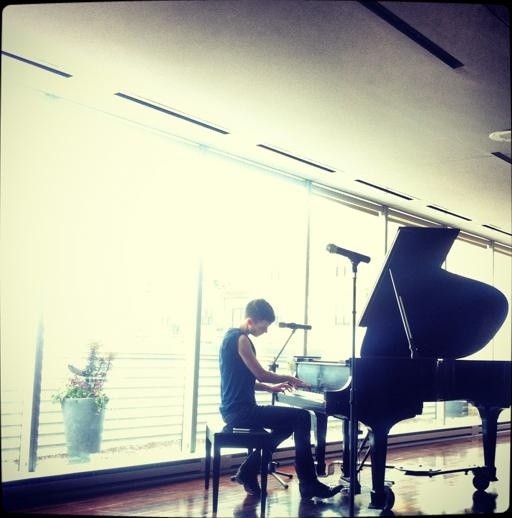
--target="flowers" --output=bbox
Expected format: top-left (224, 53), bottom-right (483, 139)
top-left (51, 340), bottom-right (114, 410)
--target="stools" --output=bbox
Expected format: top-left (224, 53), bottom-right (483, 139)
top-left (205, 425), bottom-right (271, 493)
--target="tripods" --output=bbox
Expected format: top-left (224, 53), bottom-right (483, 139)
top-left (231, 365), bottom-right (294, 489)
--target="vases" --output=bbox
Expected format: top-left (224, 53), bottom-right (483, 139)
top-left (61, 397), bottom-right (105, 464)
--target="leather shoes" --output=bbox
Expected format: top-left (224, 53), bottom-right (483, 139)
top-left (236, 470), bottom-right (261, 496)
top-left (299, 480), bottom-right (344, 499)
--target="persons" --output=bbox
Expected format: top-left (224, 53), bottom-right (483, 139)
top-left (219, 299), bottom-right (344, 500)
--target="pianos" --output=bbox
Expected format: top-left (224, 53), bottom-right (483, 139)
top-left (271, 227), bottom-right (511, 514)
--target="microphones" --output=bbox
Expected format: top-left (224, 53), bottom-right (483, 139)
top-left (325, 242), bottom-right (372, 266)
top-left (280, 322), bottom-right (313, 331)
